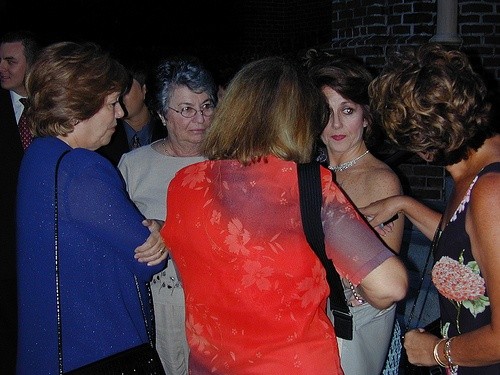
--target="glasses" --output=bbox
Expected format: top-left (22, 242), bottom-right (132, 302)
top-left (168, 105), bottom-right (214, 119)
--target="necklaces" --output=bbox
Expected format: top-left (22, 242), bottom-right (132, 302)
top-left (327, 149), bottom-right (370, 172)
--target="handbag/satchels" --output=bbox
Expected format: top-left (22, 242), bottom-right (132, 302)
top-left (64, 344), bottom-right (166, 375)
top-left (382, 314), bottom-right (411, 375)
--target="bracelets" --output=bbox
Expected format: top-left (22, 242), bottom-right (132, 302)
top-left (432, 336), bottom-right (459, 375)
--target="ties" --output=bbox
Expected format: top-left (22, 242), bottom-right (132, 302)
top-left (18, 98), bottom-right (33, 151)
top-left (132, 134), bottom-right (140, 150)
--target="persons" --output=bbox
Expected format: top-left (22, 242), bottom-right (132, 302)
top-left (355, 40), bottom-right (500, 375)
top-left (161, 56), bottom-right (409, 375)
top-left (305, 53), bottom-right (406, 375)
top-left (0, 35), bottom-right (218, 375)
